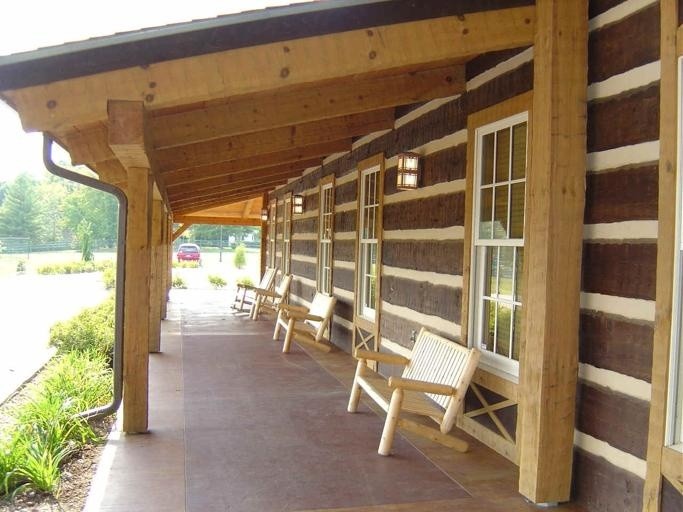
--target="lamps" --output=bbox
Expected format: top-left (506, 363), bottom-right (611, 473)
top-left (394, 148), bottom-right (420, 191)
top-left (260, 206), bottom-right (269, 222)
top-left (291, 195), bottom-right (305, 215)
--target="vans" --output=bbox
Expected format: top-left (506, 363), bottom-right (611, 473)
top-left (177, 243), bottom-right (201, 262)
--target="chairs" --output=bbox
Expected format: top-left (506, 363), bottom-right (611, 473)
top-left (231, 262), bottom-right (281, 314)
top-left (273, 287), bottom-right (337, 352)
top-left (346, 324), bottom-right (483, 459)
top-left (247, 272), bottom-right (293, 324)
